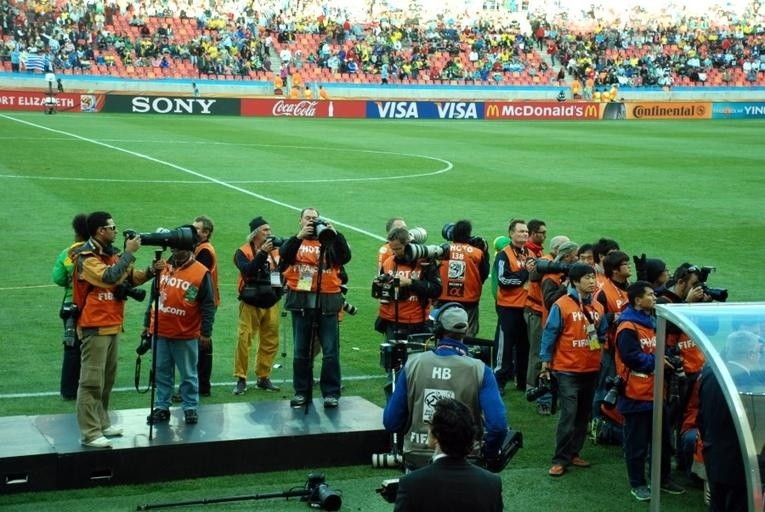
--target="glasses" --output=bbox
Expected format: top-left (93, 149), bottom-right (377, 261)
top-left (533, 231), bottom-right (547, 235)
top-left (688, 263), bottom-right (701, 273)
top-left (102, 224), bottom-right (116, 231)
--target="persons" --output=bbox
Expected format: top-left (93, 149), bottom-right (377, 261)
top-left (143, 215), bottom-right (218, 424)
top-left (373, 217), bottom-right (490, 400)
top-left (232, 216), bottom-right (284, 395)
top-left (54, 209), bottom-right (165, 448)
top-left (0, 0), bottom-right (765, 101)
top-left (279, 208), bottom-right (351, 408)
top-left (493, 219), bottom-right (764, 512)
top-left (383, 302), bottom-right (507, 474)
top-left (394, 398), bottom-right (504, 512)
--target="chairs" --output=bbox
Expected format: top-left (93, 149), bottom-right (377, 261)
top-left (0, 0), bottom-right (765, 91)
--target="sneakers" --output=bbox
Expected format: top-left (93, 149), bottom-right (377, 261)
top-left (79, 424), bottom-right (123, 449)
top-left (536, 403), bottom-right (552, 416)
top-left (184, 409), bottom-right (199, 423)
top-left (147, 406), bottom-right (171, 424)
top-left (660, 482), bottom-right (687, 496)
top-left (290, 394), bottom-right (313, 408)
top-left (323, 396), bottom-right (339, 408)
top-left (629, 485), bottom-right (653, 501)
top-left (549, 464), bottom-right (565, 477)
top-left (255, 377), bottom-right (281, 392)
top-left (571, 457), bottom-right (591, 468)
top-left (232, 378), bottom-right (249, 395)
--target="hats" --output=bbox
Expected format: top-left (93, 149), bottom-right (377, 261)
top-left (248, 216), bottom-right (269, 232)
top-left (429, 302), bottom-right (469, 334)
top-left (632, 253), bottom-right (666, 283)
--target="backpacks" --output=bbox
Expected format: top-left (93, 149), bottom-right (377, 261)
top-left (237, 240), bottom-right (283, 308)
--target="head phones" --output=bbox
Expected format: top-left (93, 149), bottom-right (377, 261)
top-left (432, 302), bottom-right (468, 340)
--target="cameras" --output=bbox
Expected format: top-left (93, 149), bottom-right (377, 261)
top-left (115, 279), bottom-right (146, 304)
top-left (60, 302), bottom-right (83, 348)
top-left (375, 272), bottom-right (392, 284)
top-left (696, 266), bottom-right (728, 302)
top-left (137, 332), bottom-right (151, 354)
top-left (526, 375), bottom-right (553, 401)
top-left (308, 470), bottom-right (343, 509)
top-left (604, 375), bottom-right (624, 406)
top-left (265, 234), bottom-right (288, 248)
